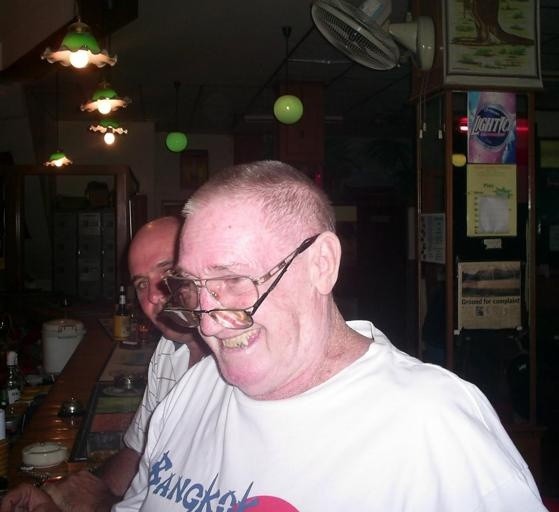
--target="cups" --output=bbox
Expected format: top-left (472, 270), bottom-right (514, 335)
top-left (0, 411), bottom-right (7, 441)
top-left (120, 320), bottom-right (151, 347)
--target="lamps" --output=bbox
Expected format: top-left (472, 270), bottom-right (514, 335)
top-left (81, 65), bottom-right (136, 116)
top-left (270, 27), bottom-right (310, 125)
top-left (38, 0), bottom-right (120, 70)
top-left (164, 79), bottom-right (191, 154)
top-left (43, 72), bottom-right (77, 172)
top-left (88, 116), bottom-right (130, 146)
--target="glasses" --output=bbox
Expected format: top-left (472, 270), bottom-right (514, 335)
top-left (161, 233), bottom-right (319, 331)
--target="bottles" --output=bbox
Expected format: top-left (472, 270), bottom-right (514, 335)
top-left (0, 347), bottom-right (28, 430)
top-left (113, 284), bottom-right (129, 341)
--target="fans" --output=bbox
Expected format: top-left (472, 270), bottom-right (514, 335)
top-left (310, 0), bottom-right (437, 76)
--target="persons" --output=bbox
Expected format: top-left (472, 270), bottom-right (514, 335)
top-left (104, 159), bottom-right (551, 512)
top-left (0, 215), bottom-right (215, 512)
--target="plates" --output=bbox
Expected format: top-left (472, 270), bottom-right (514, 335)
top-left (102, 386), bottom-right (144, 397)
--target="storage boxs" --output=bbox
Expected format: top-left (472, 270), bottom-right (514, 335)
top-left (51, 210), bottom-right (116, 303)
